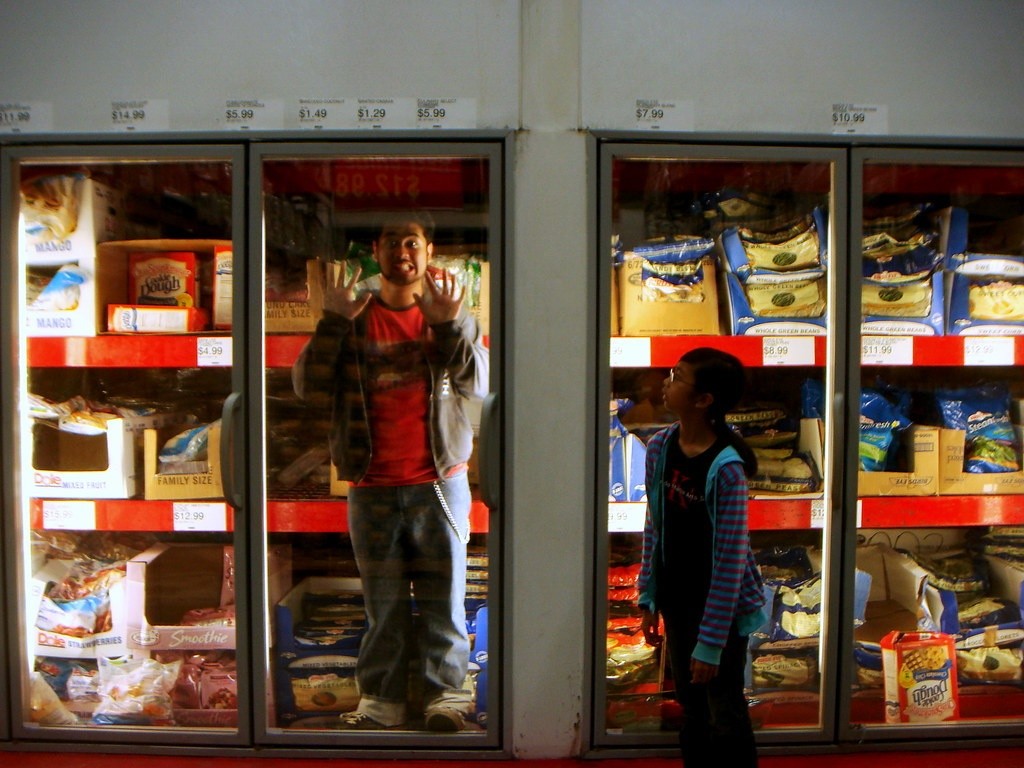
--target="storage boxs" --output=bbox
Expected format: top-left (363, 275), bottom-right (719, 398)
top-left (882, 631), bottom-right (959, 724)
top-left (265, 257), bottom-right (328, 335)
top-left (98, 238), bottom-right (234, 336)
top-left (276, 575), bottom-right (489, 711)
top-left (18, 177), bottom-right (127, 338)
top-left (858, 398), bottom-right (1024, 495)
top-left (108, 245), bottom-right (234, 332)
top-left (32, 545), bottom-right (236, 658)
top-left (29, 396), bottom-right (224, 502)
top-left (853, 543), bottom-right (1024, 651)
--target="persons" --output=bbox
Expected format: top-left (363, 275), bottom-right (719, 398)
top-left (635, 349), bottom-right (770, 768)
top-left (291, 208), bottom-right (477, 731)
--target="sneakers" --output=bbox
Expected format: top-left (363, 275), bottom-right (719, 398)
top-left (425, 706), bottom-right (466, 731)
top-left (337, 712), bottom-right (383, 729)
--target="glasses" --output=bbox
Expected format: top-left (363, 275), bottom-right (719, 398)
top-left (670, 368), bottom-right (696, 388)
